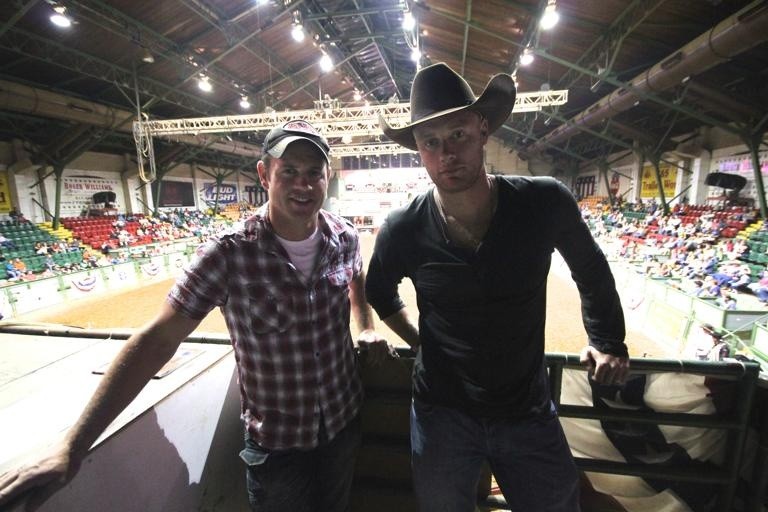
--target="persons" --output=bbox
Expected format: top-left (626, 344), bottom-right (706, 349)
top-left (578, 199), bottom-right (768, 361)
top-left (364, 63), bottom-right (630, 511)
top-left (0, 202), bottom-right (254, 284)
top-left (0, 118), bottom-right (402, 512)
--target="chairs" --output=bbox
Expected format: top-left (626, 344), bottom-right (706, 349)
top-left (650, 204), bottom-right (768, 287)
top-left (0, 213), bottom-right (153, 279)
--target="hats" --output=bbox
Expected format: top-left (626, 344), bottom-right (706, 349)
top-left (260, 120), bottom-right (332, 168)
top-left (378, 62), bottom-right (516, 151)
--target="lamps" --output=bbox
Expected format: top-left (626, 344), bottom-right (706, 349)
top-left (240, 96), bottom-right (249, 109)
top-left (520, 48), bottom-right (534, 65)
top-left (291, 11), bottom-right (304, 42)
top-left (51, 3), bottom-right (71, 26)
top-left (142, 49), bottom-right (154, 63)
top-left (200, 76), bottom-right (212, 92)
